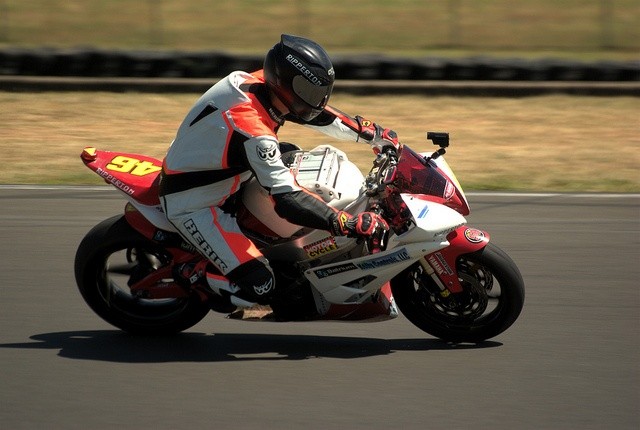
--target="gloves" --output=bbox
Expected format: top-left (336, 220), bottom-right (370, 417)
top-left (327, 210), bottom-right (389, 240)
top-left (356, 114), bottom-right (399, 153)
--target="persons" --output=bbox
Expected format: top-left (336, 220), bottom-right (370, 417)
top-left (158, 34), bottom-right (401, 308)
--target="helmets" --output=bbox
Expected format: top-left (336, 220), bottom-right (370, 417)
top-left (263, 34), bottom-right (335, 121)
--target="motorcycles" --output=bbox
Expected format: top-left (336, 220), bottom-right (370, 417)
top-left (74, 130), bottom-right (525, 342)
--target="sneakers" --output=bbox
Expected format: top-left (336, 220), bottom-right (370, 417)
top-left (174, 261), bottom-right (238, 313)
